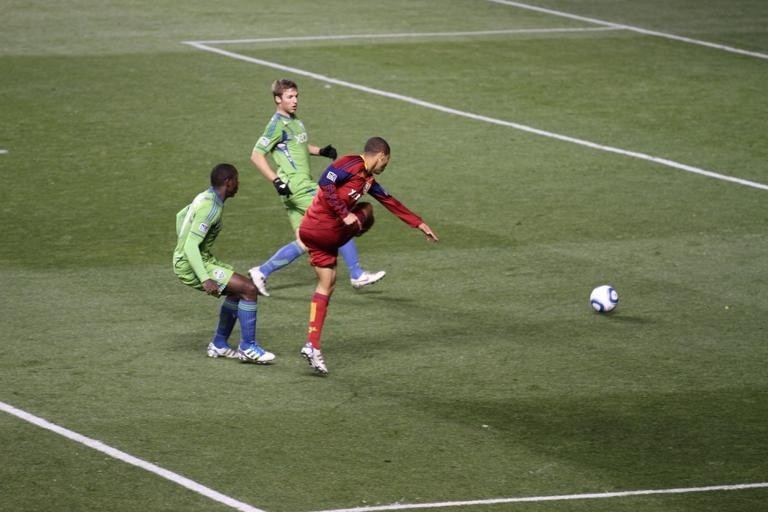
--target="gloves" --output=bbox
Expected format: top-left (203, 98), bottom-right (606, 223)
top-left (317, 143), bottom-right (338, 162)
top-left (272, 177), bottom-right (293, 198)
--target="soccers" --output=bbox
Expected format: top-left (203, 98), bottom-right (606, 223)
top-left (590, 285), bottom-right (618, 313)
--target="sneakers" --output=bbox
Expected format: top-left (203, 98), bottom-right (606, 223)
top-left (348, 268), bottom-right (387, 289)
top-left (236, 341), bottom-right (276, 366)
top-left (206, 341), bottom-right (242, 363)
top-left (301, 343), bottom-right (330, 376)
top-left (247, 266), bottom-right (271, 298)
top-left (355, 217), bottom-right (374, 237)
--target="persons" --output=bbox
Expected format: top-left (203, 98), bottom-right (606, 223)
top-left (246, 76), bottom-right (387, 298)
top-left (171, 162), bottom-right (277, 364)
top-left (298, 135), bottom-right (439, 377)
